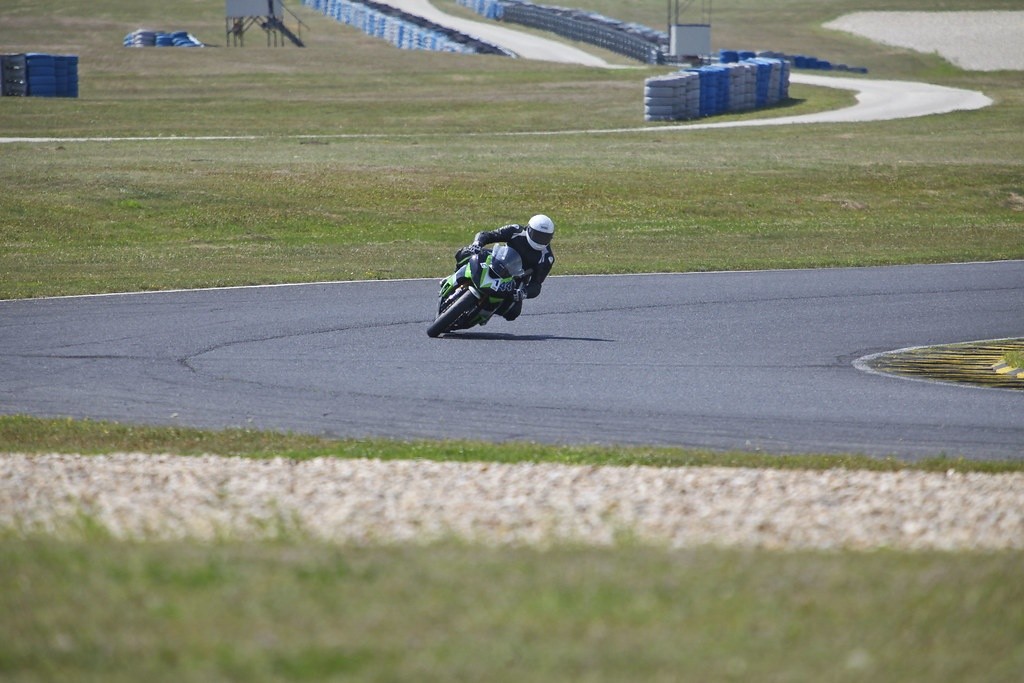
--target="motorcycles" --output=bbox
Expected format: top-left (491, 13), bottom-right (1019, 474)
top-left (427, 243), bottom-right (535, 337)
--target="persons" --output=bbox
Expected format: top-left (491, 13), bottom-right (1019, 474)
top-left (471, 214), bottom-right (556, 325)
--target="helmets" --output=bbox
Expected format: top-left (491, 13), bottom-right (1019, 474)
top-left (526, 215), bottom-right (555, 250)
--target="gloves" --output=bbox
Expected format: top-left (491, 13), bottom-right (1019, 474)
top-left (513, 288), bottom-right (527, 301)
top-left (468, 245), bottom-right (480, 253)
